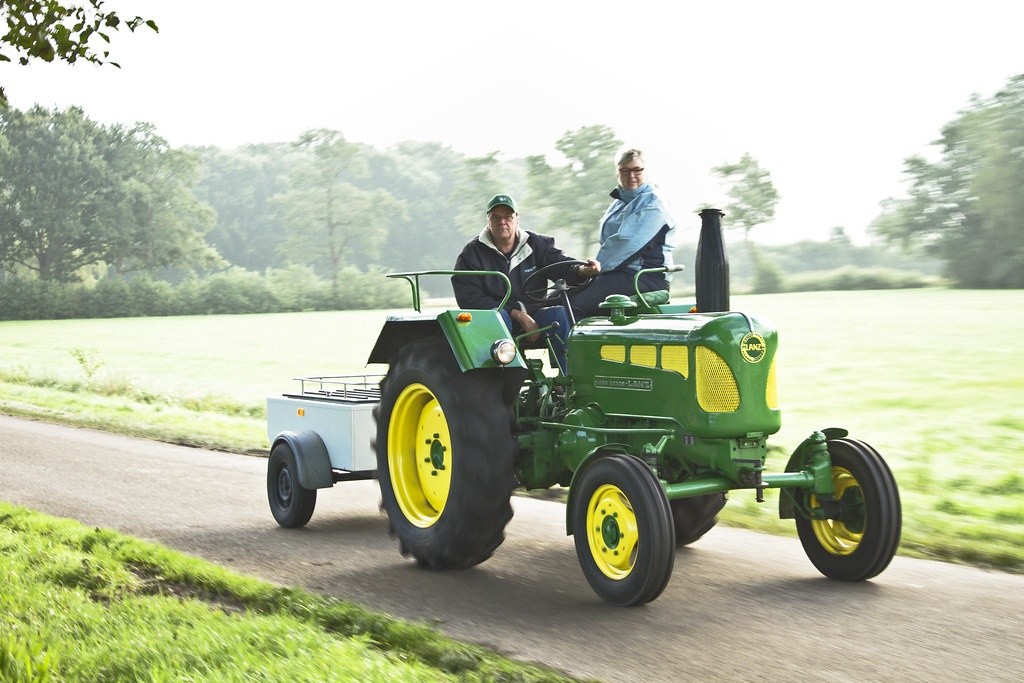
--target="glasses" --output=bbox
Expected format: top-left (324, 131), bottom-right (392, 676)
top-left (488, 214), bottom-right (516, 221)
top-left (619, 167), bottom-right (644, 175)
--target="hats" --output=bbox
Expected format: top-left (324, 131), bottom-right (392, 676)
top-left (486, 194), bottom-right (517, 214)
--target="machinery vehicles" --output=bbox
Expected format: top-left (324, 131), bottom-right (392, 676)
top-left (262, 206), bottom-right (902, 607)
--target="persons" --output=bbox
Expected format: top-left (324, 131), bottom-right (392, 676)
top-left (547, 146), bottom-right (674, 328)
top-left (451, 195), bottom-right (602, 394)
